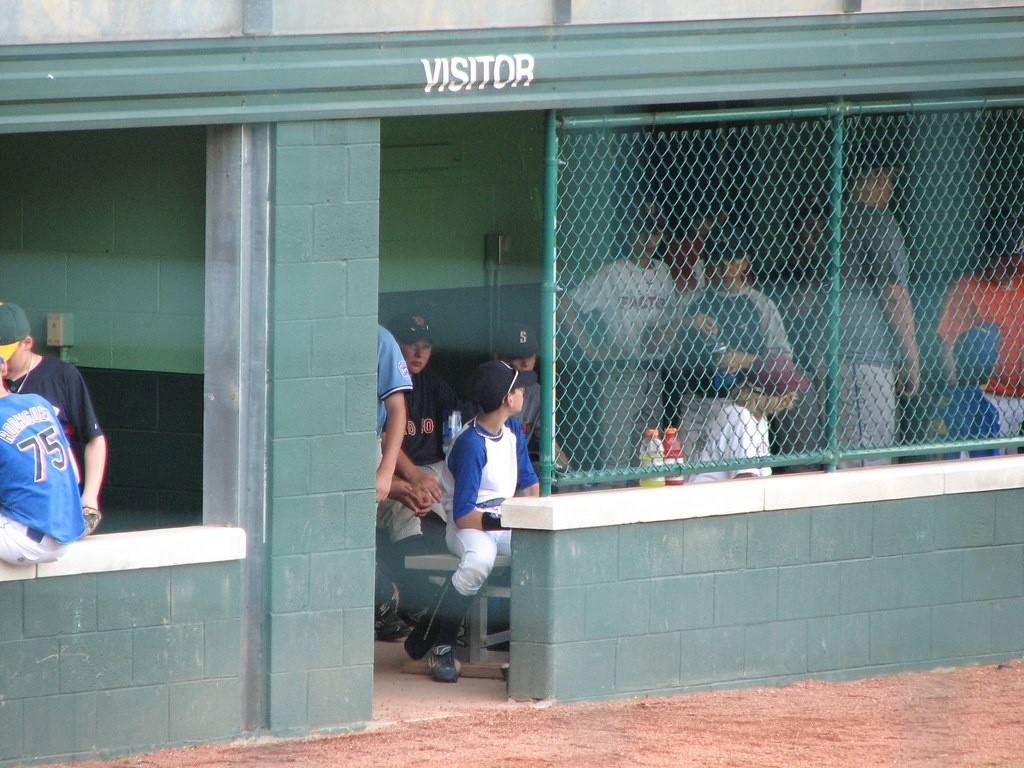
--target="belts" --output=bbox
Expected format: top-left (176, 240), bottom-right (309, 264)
top-left (613, 359), bottom-right (661, 372)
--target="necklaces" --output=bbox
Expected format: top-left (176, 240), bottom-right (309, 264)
top-left (474, 418), bottom-right (502, 439)
top-left (4, 358), bottom-right (32, 393)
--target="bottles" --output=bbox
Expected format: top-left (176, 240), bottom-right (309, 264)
top-left (639, 429), bottom-right (666, 487)
top-left (704, 329), bottom-right (737, 389)
top-left (442, 411), bottom-right (462, 455)
top-left (662, 428), bottom-right (685, 485)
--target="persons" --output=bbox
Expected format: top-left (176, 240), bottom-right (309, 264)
top-left (641, 197), bottom-right (795, 481)
top-left (936, 221), bottom-right (1024, 456)
top-left (0, 302), bottom-right (106, 536)
top-left (785, 147), bottom-right (920, 474)
top-left (0, 359), bottom-right (84, 566)
top-left (462, 325), bottom-right (541, 449)
top-left (389, 310), bottom-right (463, 624)
top-left (562, 197), bottom-right (677, 488)
top-left (925, 323), bottom-right (1001, 463)
top-left (377, 324), bottom-right (414, 629)
top-left (425, 360), bottom-right (539, 683)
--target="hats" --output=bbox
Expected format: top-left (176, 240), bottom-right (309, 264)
top-left (843, 144), bottom-right (908, 174)
top-left (744, 350), bottom-right (810, 395)
top-left (387, 313), bottom-right (434, 343)
top-left (977, 208), bottom-right (1024, 257)
top-left (951, 323), bottom-right (1001, 376)
top-left (633, 203), bottom-right (667, 227)
top-left (492, 322), bottom-right (538, 357)
top-left (0, 302), bottom-right (31, 345)
top-left (474, 359), bottom-right (538, 401)
top-left (703, 228), bottom-right (752, 258)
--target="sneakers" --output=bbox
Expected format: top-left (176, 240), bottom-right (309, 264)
top-left (426, 644), bottom-right (460, 683)
top-left (404, 614), bottom-right (441, 658)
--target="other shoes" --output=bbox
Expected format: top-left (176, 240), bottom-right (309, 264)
top-left (374, 611), bottom-right (412, 641)
top-left (406, 606), bottom-right (465, 637)
top-left (378, 582), bottom-right (399, 616)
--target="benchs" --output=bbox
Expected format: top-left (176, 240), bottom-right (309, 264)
top-left (402, 553), bottom-right (511, 678)
